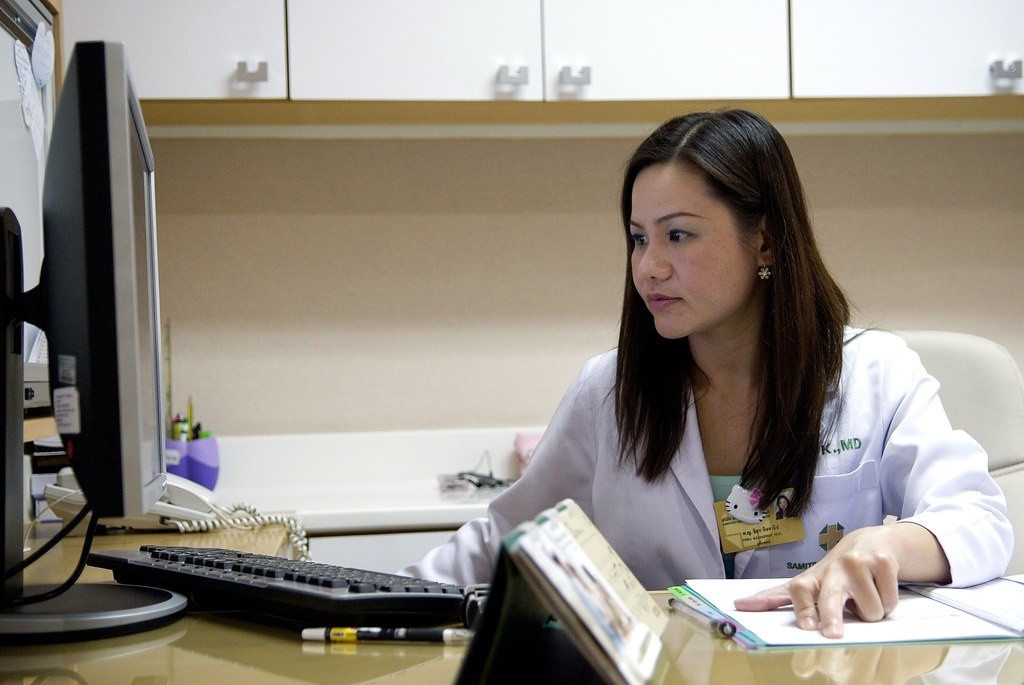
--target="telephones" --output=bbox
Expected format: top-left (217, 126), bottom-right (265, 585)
top-left (44, 467), bottom-right (221, 537)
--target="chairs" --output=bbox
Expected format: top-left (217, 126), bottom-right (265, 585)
top-left (887, 328), bottom-right (1024, 580)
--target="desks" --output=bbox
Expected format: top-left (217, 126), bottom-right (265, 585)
top-left (0, 510), bottom-right (1024, 685)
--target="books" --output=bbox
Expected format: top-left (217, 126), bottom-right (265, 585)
top-left (668, 572), bottom-right (1024, 646)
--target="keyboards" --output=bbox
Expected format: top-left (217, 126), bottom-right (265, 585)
top-left (84, 545), bottom-right (467, 635)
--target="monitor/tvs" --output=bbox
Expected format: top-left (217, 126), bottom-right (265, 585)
top-left (0, 41), bottom-right (188, 644)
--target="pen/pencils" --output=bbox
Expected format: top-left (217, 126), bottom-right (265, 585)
top-left (172, 394), bottom-right (203, 440)
top-left (300, 626), bottom-right (471, 644)
top-left (668, 597), bottom-right (737, 639)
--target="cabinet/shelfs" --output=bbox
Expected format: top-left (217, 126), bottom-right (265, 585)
top-left (45, 0), bottom-right (1024, 136)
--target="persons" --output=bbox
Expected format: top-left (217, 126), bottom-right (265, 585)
top-left (383, 108), bottom-right (1017, 638)
